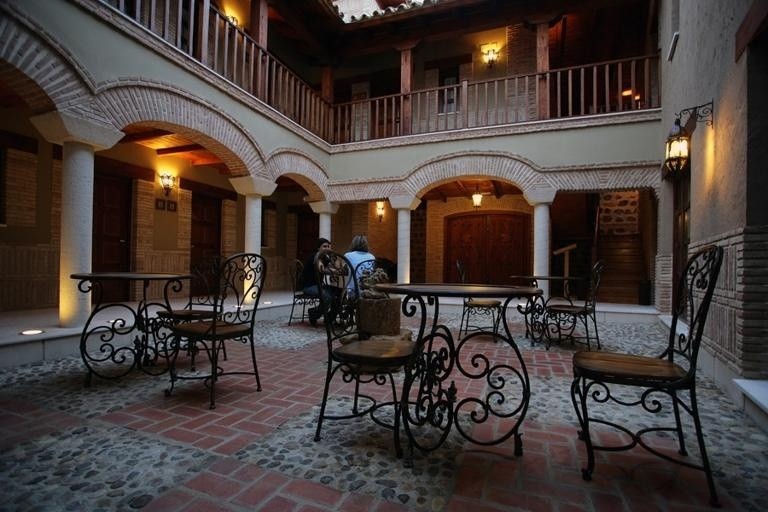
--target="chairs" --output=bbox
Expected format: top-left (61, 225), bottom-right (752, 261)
top-left (164, 253), bottom-right (268, 409)
top-left (314, 250), bottom-right (419, 452)
top-left (288, 260), bottom-right (323, 324)
top-left (456, 259), bottom-right (504, 342)
top-left (333, 259), bottom-right (388, 332)
top-left (156, 254), bottom-right (231, 370)
top-left (576, 243), bottom-right (727, 477)
top-left (546, 262), bottom-right (601, 348)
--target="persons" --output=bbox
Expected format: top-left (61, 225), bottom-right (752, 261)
top-left (297, 236), bottom-right (347, 328)
top-left (341, 233), bottom-right (376, 319)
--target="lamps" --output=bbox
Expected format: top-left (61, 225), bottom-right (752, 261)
top-left (663, 101), bottom-right (713, 175)
top-left (485, 49), bottom-right (500, 69)
top-left (229, 14), bottom-right (238, 31)
top-left (376, 200), bottom-right (387, 223)
top-left (471, 191), bottom-right (482, 207)
top-left (160, 172), bottom-right (175, 196)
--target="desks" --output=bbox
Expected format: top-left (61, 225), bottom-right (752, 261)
top-left (65, 272), bottom-right (195, 380)
top-left (324, 270), bottom-right (348, 277)
top-left (374, 285), bottom-right (542, 472)
top-left (510, 274), bottom-right (577, 338)
